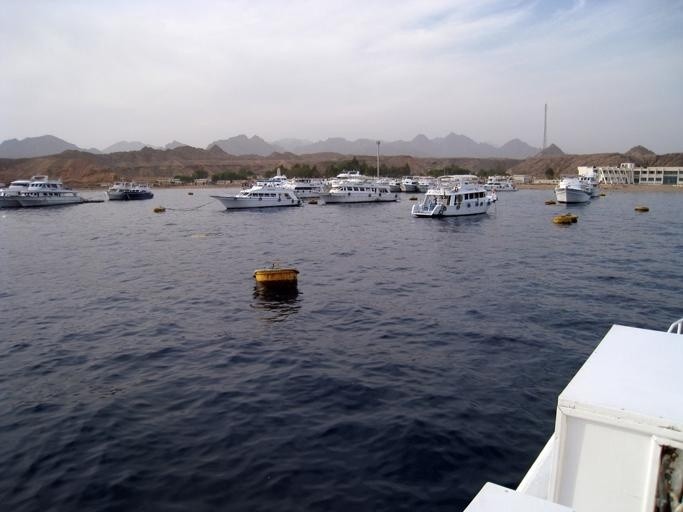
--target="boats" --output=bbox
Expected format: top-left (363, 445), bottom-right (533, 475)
top-left (401, 175), bottom-right (514, 191)
top-left (411, 187), bottom-right (497, 216)
top-left (108, 182), bottom-right (154, 201)
top-left (0, 176), bottom-right (83, 208)
top-left (211, 170), bottom-right (398, 203)
top-left (554, 175), bottom-right (601, 204)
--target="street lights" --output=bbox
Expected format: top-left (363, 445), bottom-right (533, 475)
top-left (376, 139), bottom-right (381, 176)
